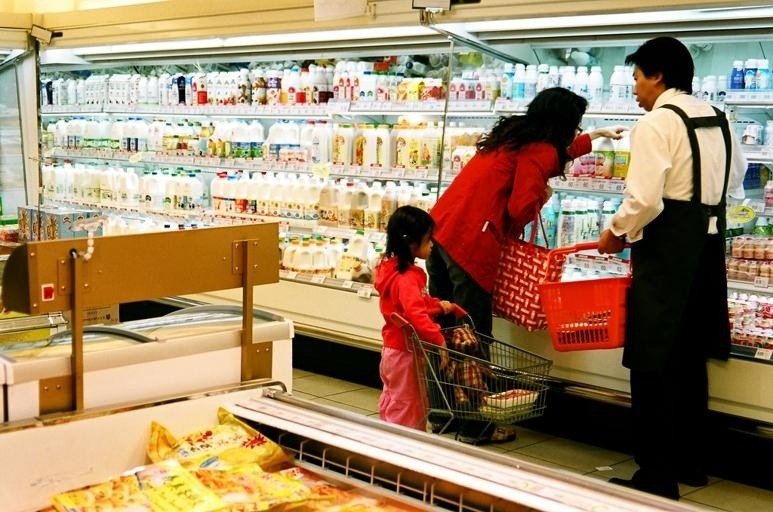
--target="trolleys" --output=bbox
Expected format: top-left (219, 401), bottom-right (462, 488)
top-left (392, 303), bottom-right (552, 446)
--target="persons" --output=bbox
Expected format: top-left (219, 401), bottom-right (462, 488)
top-left (371, 204), bottom-right (455, 434)
top-left (594, 34), bottom-right (750, 503)
top-left (423, 86), bottom-right (632, 447)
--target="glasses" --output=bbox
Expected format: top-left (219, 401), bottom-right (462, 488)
top-left (575, 125), bottom-right (583, 136)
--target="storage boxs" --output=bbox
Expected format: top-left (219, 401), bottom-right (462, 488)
top-left (16, 202), bottom-right (105, 246)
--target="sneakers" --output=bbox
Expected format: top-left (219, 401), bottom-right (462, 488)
top-left (608, 477), bottom-right (680, 501)
top-left (680, 470), bottom-right (711, 488)
top-left (430, 418), bottom-right (517, 445)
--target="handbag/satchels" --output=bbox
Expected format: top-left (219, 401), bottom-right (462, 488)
top-left (492, 199), bottom-right (564, 332)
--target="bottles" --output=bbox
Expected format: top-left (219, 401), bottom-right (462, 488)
top-left (501, 62), bottom-right (634, 103)
top-left (762, 120), bottom-right (772, 144)
top-left (40, 61), bottom-right (497, 228)
top-left (690, 58), bottom-right (770, 101)
top-left (529, 192), bottom-right (619, 248)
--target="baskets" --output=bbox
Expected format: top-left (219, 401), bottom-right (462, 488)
top-left (535, 241), bottom-right (634, 353)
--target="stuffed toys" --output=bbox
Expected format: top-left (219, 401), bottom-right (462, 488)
top-left (443, 324), bottom-right (494, 410)
